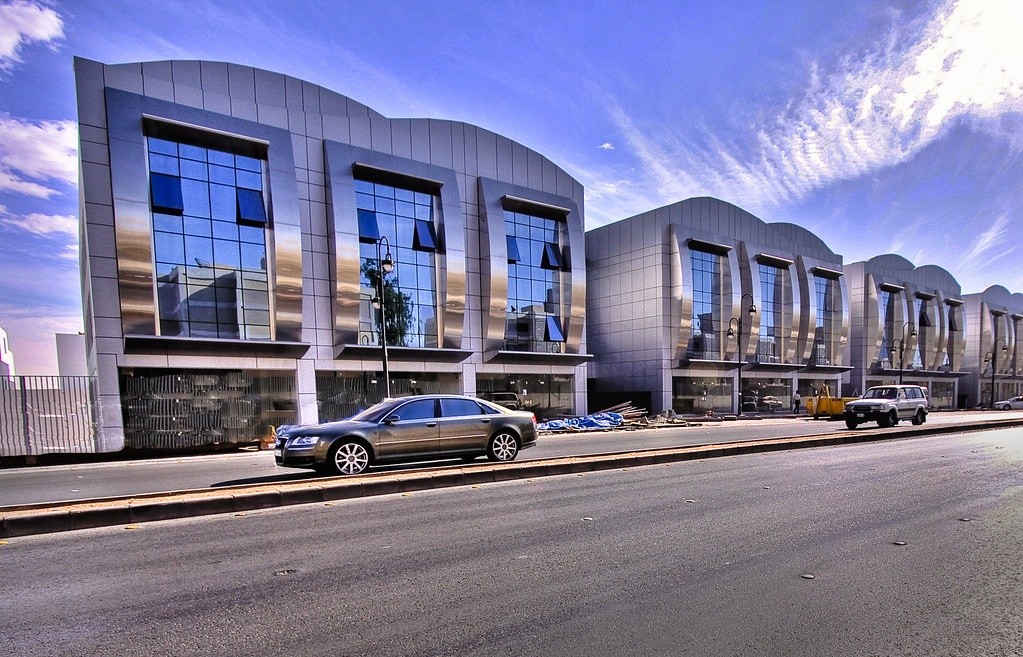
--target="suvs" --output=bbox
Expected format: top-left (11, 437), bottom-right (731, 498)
top-left (843, 385), bottom-right (929, 429)
top-left (478, 393), bottom-right (520, 411)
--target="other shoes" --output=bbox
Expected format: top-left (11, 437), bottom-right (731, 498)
top-left (797, 413), bottom-right (800, 414)
top-left (793, 411), bottom-right (795, 414)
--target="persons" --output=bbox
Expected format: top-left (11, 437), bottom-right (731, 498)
top-left (793, 391), bottom-right (801, 414)
top-left (978, 401), bottom-right (984, 407)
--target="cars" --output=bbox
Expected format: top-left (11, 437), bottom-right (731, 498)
top-left (992, 396), bottom-right (1023, 411)
top-left (274, 394), bottom-right (538, 475)
top-left (744, 396), bottom-right (757, 411)
top-left (759, 396), bottom-right (783, 409)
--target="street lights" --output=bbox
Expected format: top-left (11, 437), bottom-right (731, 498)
top-left (727, 293), bottom-right (756, 413)
top-left (890, 322), bottom-right (917, 385)
top-left (371, 235), bottom-right (396, 398)
top-left (984, 339), bottom-right (1008, 408)
